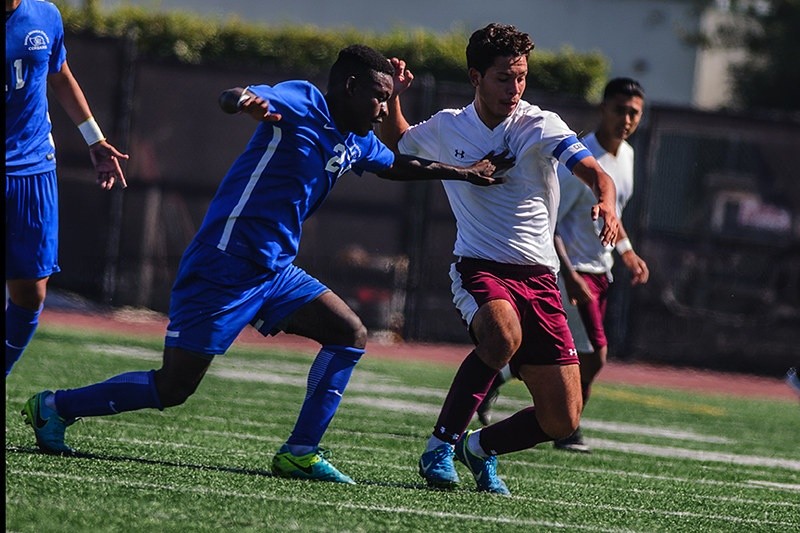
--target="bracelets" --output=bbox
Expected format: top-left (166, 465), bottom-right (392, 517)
top-left (78, 117), bottom-right (106, 146)
top-left (616, 238), bottom-right (632, 255)
top-left (237, 94), bottom-right (250, 105)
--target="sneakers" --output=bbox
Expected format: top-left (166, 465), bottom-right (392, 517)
top-left (455, 430), bottom-right (510, 495)
top-left (21, 390), bottom-right (83, 457)
top-left (271, 442), bottom-right (356, 485)
top-left (419, 441), bottom-right (460, 489)
top-left (554, 427), bottom-right (588, 453)
top-left (477, 387), bottom-right (500, 425)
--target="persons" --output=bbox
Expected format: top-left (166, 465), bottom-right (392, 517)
top-left (380, 21), bottom-right (621, 494)
top-left (1, 0), bottom-right (129, 380)
top-left (21, 44), bottom-right (517, 485)
top-left (475, 77), bottom-right (650, 454)
